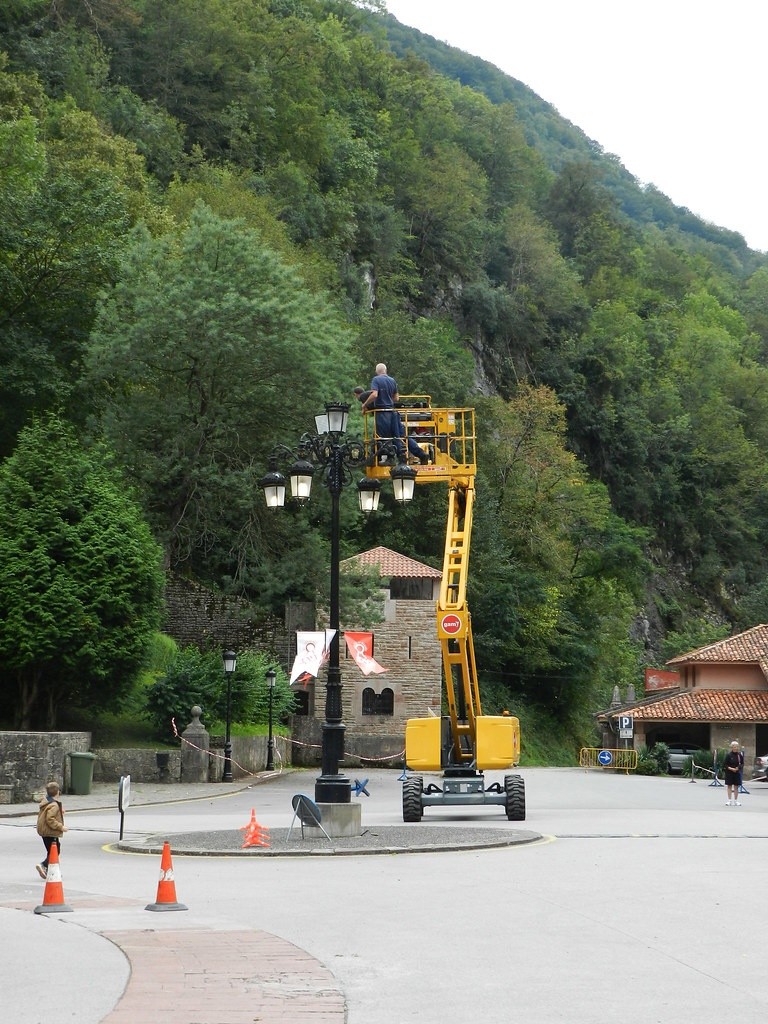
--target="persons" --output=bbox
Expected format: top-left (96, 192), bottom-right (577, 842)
top-left (354, 363), bottom-right (430, 465)
top-left (36, 782), bottom-right (69, 878)
top-left (725, 741), bottom-right (744, 806)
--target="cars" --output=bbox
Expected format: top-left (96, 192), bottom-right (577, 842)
top-left (649, 742), bottom-right (708, 774)
top-left (756, 753), bottom-right (768, 781)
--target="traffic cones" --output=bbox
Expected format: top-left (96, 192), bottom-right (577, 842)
top-left (144, 842), bottom-right (188, 912)
top-left (34, 836), bottom-right (75, 915)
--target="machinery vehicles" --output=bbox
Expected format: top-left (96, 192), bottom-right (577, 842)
top-left (365, 396), bottom-right (526, 822)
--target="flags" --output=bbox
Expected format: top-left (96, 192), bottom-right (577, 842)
top-left (290, 629), bottom-right (336, 689)
top-left (344, 632), bottom-right (391, 675)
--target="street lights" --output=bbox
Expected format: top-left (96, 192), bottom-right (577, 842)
top-left (219, 650), bottom-right (237, 781)
top-left (262, 667), bottom-right (278, 771)
top-left (260, 401), bottom-right (420, 839)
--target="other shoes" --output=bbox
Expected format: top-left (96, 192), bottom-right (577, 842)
top-left (36, 863), bottom-right (47, 879)
top-left (725, 801), bottom-right (733, 806)
top-left (734, 801), bottom-right (741, 806)
top-left (379, 458), bottom-right (396, 466)
top-left (421, 454), bottom-right (429, 465)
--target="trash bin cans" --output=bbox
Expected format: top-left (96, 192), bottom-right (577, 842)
top-left (67, 752), bottom-right (98, 795)
